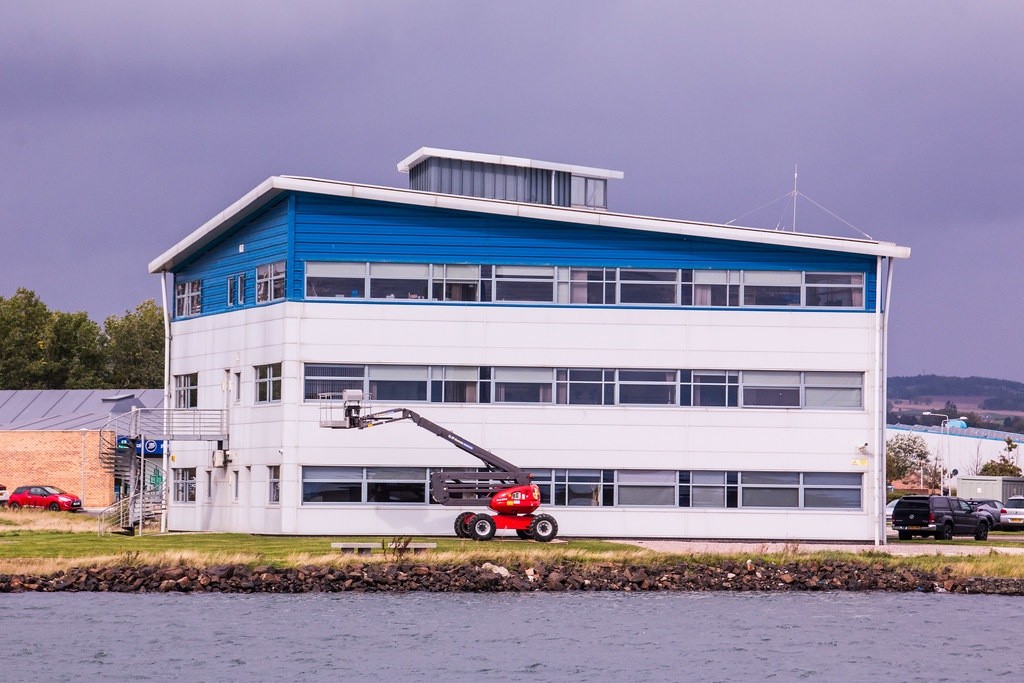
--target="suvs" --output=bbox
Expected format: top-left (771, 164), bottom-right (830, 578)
top-left (999, 494), bottom-right (1024, 531)
top-left (891, 493), bottom-right (989, 541)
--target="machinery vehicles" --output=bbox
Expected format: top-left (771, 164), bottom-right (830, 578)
top-left (316, 390), bottom-right (560, 543)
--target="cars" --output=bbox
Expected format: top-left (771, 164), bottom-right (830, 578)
top-left (8, 484), bottom-right (82, 514)
top-left (886, 498), bottom-right (899, 520)
top-left (960, 496), bottom-right (1005, 531)
top-left (0, 484), bottom-right (8, 507)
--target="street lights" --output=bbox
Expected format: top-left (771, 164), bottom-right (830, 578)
top-left (923, 412), bottom-right (953, 496)
top-left (78, 427), bottom-right (89, 507)
top-left (940, 416), bottom-right (968, 496)
